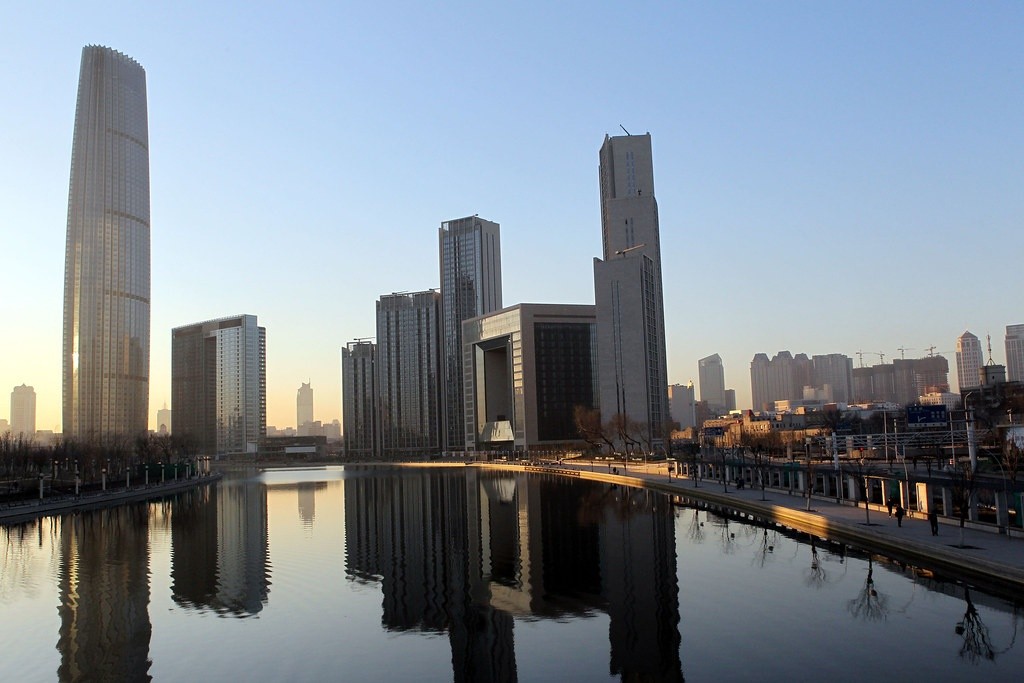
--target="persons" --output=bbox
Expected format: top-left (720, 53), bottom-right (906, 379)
top-left (886, 498), bottom-right (894, 517)
top-left (928, 509), bottom-right (938, 536)
top-left (896, 503), bottom-right (904, 527)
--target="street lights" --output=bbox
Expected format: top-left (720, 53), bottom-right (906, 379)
top-left (145, 466), bottom-right (149, 485)
top-left (875, 445), bottom-right (910, 511)
top-left (161, 465), bottom-right (164, 483)
top-left (185, 462), bottom-right (189, 479)
top-left (630, 446), bottom-right (646, 469)
top-left (126, 467), bottom-right (130, 488)
top-left (39, 473), bottom-right (45, 505)
top-left (75, 470), bottom-right (80, 495)
top-left (102, 468), bottom-right (106, 490)
top-left (54, 460), bottom-right (58, 478)
top-left (174, 464), bottom-right (177, 481)
top-left (74, 460), bottom-right (78, 471)
top-left (107, 458), bottom-right (110, 476)
